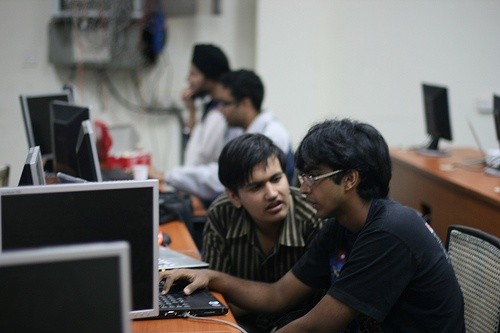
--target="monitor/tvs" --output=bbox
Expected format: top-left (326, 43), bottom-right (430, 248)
top-left (418, 82), bottom-right (452, 158)
top-left (0, 88), bottom-right (158, 332)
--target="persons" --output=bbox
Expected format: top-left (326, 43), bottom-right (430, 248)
top-left (147, 68), bottom-right (295, 202)
top-left (159, 118), bottom-right (465, 333)
top-left (200, 132), bottom-right (328, 333)
top-left (180, 44), bottom-right (231, 150)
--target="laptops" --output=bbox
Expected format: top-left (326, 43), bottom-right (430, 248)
top-left (158, 277), bottom-right (229, 317)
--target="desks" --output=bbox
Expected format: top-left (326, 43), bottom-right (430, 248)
top-left (387, 147), bottom-right (500, 249)
top-left (130, 171), bottom-right (243, 333)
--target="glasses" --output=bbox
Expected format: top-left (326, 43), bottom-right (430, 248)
top-left (297, 168), bottom-right (342, 187)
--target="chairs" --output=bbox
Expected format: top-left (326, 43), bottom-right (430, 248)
top-left (445, 225), bottom-right (500, 333)
